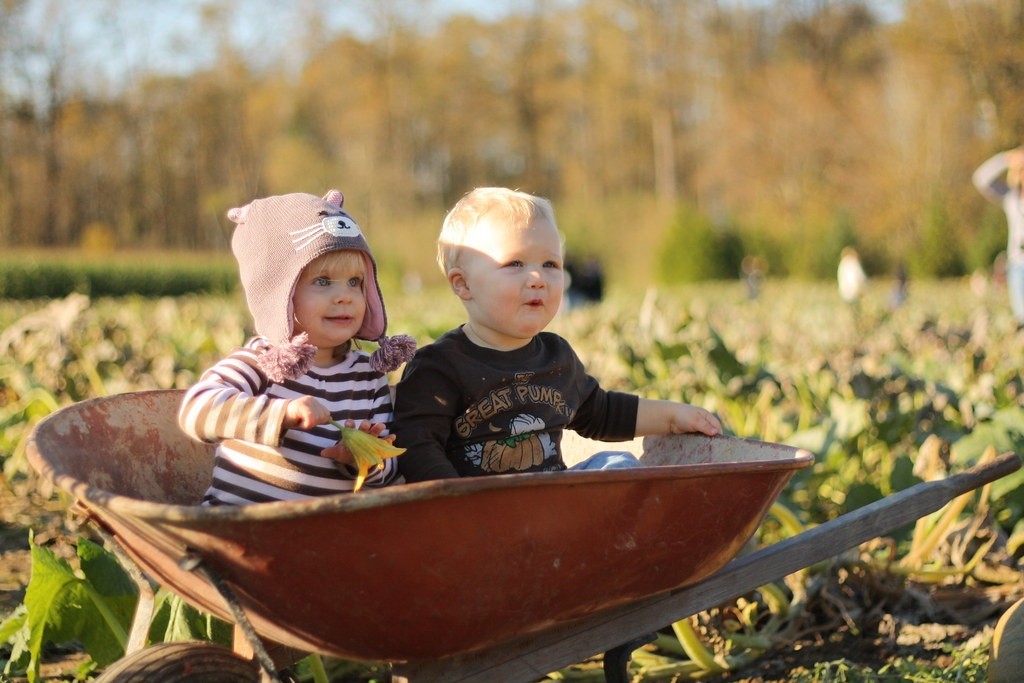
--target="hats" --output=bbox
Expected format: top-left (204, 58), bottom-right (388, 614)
top-left (227, 189), bottom-right (416, 382)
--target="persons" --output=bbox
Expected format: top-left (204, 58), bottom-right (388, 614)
top-left (178, 190), bottom-right (417, 506)
top-left (393, 187), bottom-right (723, 484)
top-left (973, 147), bottom-right (1024, 331)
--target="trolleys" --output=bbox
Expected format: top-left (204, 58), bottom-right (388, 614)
top-left (22, 389), bottom-right (1024, 683)
